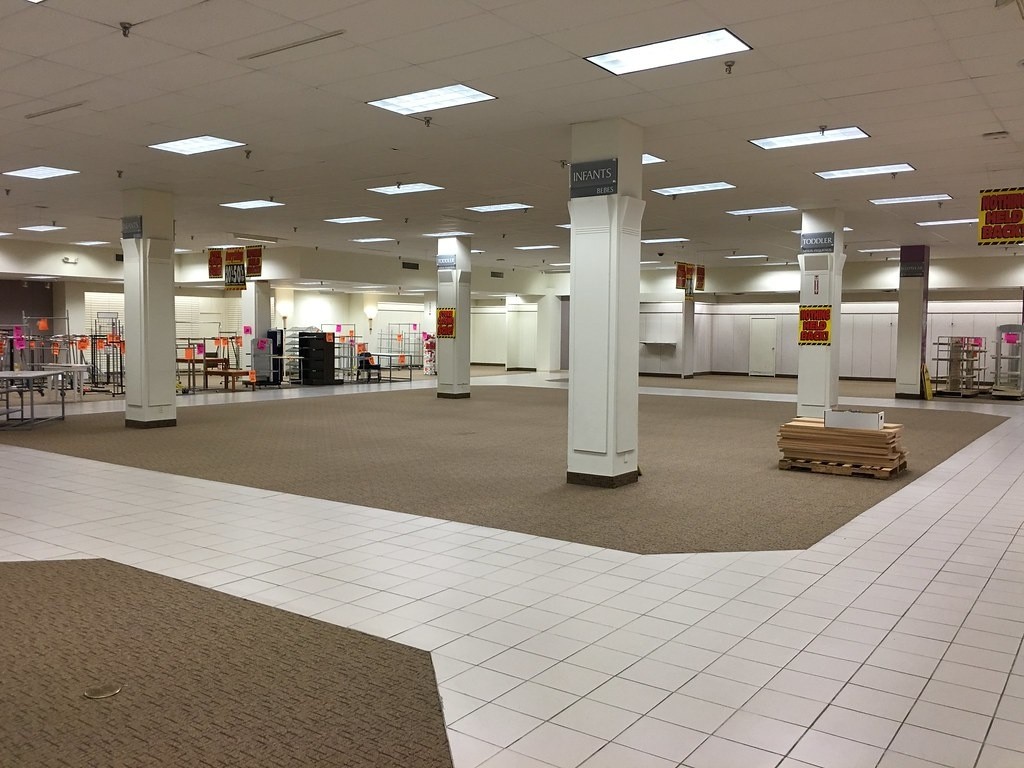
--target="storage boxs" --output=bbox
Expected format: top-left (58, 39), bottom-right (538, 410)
top-left (824, 409), bottom-right (884, 431)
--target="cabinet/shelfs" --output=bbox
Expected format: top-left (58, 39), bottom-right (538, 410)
top-left (1, 311), bottom-right (437, 431)
top-left (929, 324), bottom-right (1024, 398)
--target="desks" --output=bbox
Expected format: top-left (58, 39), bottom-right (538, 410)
top-left (205, 368), bottom-right (250, 391)
top-left (1, 370), bottom-right (66, 428)
top-left (27, 361), bottom-right (91, 402)
top-left (176, 357), bottom-right (229, 389)
top-left (369, 352), bottom-right (412, 381)
